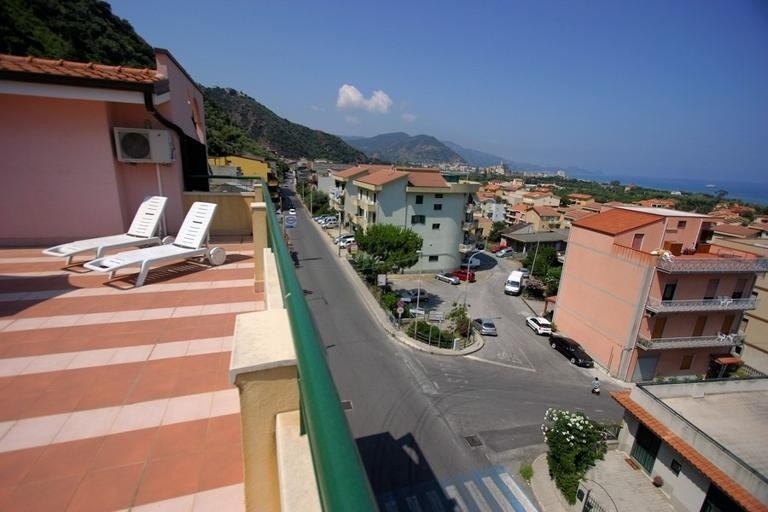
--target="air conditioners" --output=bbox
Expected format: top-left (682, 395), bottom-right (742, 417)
top-left (114, 126), bottom-right (175, 166)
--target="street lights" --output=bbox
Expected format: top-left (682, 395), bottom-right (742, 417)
top-left (464, 250), bottom-right (486, 307)
top-left (415, 250), bottom-right (423, 340)
top-left (307, 183), bottom-right (313, 217)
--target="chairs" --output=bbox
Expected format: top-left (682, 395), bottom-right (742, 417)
top-left (42, 196), bottom-right (227, 289)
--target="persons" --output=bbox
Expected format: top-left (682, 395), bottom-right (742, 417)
top-left (591, 375), bottom-right (600, 394)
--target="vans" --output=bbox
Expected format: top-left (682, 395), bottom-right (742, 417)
top-left (504, 270), bottom-right (524, 296)
top-left (452, 269), bottom-right (475, 282)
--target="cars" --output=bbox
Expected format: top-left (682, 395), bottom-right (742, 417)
top-left (518, 268), bottom-right (530, 279)
top-left (549, 335), bottom-right (594, 368)
top-left (526, 317), bottom-right (552, 335)
top-left (491, 246), bottom-right (514, 258)
top-left (435, 272), bottom-right (460, 285)
top-left (473, 318), bottom-right (497, 336)
top-left (333, 234), bottom-right (357, 249)
top-left (400, 289), bottom-right (429, 303)
top-left (288, 208), bottom-right (296, 216)
top-left (313, 214), bottom-right (338, 229)
